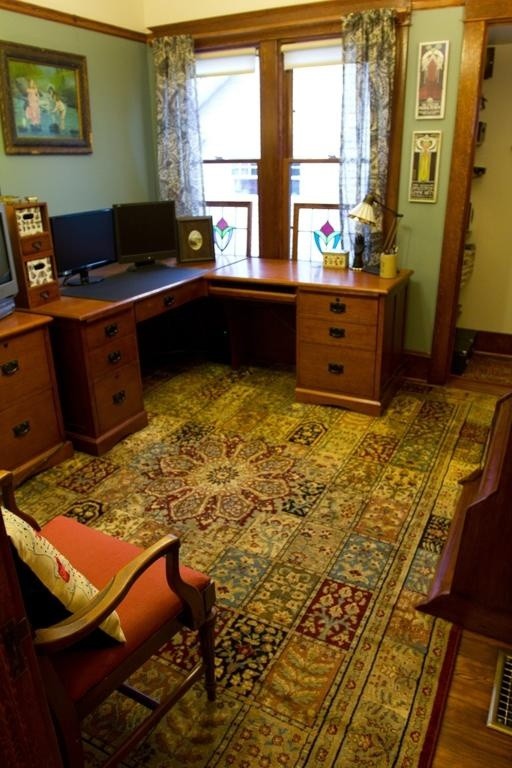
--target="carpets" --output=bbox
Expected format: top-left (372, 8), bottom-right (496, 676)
top-left (450, 354), bottom-right (512, 386)
top-left (3, 356), bottom-right (501, 767)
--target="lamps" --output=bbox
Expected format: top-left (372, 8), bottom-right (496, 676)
top-left (347, 195), bottom-right (404, 255)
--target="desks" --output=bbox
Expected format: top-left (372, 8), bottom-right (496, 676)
top-left (13, 257), bottom-right (235, 459)
top-left (205, 256), bottom-right (416, 417)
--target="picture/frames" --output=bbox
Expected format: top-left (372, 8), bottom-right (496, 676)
top-left (414, 40), bottom-right (450, 120)
top-left (0, 40), bottom-right (93, 155)
top-left (408, 131), bottom-right (442, 203)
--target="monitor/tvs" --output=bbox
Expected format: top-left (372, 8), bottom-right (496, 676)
top-left (0, 202), bottom-right (19, 319)
top-left (112, 200), bottom-right (179, 273)
top-left (49, 208), bottom-right (118, 287)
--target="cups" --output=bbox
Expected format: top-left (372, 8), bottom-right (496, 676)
top-left (380, 253), bottom-right (397, 280)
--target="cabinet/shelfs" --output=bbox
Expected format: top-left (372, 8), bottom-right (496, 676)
top-left (0, 311), bottom-right (74, 488)
top-left (4, 202), bottom-right (61, 309)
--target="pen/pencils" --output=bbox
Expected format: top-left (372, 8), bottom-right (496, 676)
top-left (384, 249), bottom-right (389, 254)
top-left (392, 244), bottom-right (395, 248)
top-left (393, 246), bottom-right (399, 253)
top-left (389, 247), bottom-right (394, 254)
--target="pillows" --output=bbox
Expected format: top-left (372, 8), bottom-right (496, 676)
top-left (1, 506), bottom-right (134, 647)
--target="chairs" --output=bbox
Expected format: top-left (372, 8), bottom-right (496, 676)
top-left (0, 469), bottom-right (217, 767)
